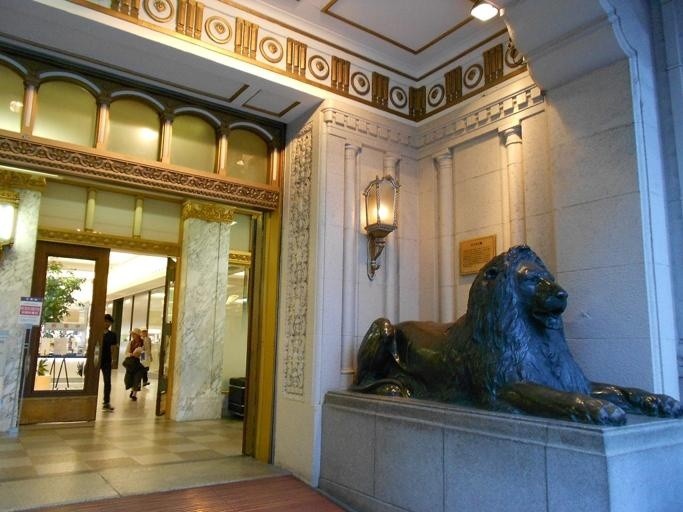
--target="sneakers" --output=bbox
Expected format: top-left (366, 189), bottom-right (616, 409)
top-left (130, 391), bottom-right (136, 401)
top-left (104, 405), bottom-right (113, 410)
top-left (143, 383), bottom-right (149, 386)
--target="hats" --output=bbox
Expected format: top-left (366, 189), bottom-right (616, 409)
top-left (130, 328), bottom-right (141, 335)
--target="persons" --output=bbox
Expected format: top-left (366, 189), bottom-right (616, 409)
top-left (140, 330), bottom-right (153, 386)
top-left (99, 313), bottom-right (117, 411)
top-left (124, 327), bottom-right (144, 401)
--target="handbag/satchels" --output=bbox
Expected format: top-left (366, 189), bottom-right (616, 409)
top-left (123, 357), bottom-right (140, 372)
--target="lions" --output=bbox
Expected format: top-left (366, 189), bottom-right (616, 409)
top-left (346, 243), bottom-right (683, 426)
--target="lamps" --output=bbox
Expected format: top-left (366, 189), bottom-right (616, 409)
top-left (358, 173), bottom-right (401, 283)
top-left (0, 186), bottom-right (20, 253)
top-left (470, 0), bottom-right (499, 22)
